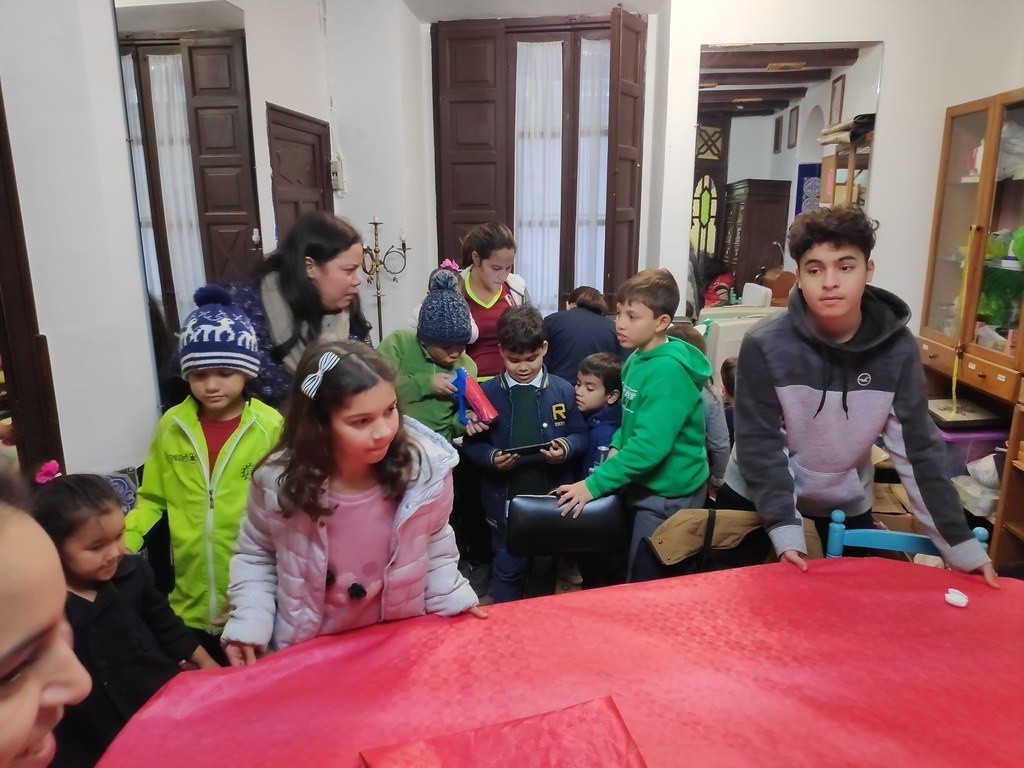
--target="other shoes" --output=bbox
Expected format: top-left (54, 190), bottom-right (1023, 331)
top-left (468, 564), bottom-right (489, 596)
top-left (561, 565), bottom-right (584, 585)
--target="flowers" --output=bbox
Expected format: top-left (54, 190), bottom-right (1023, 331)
top-left (439, 259), bottom-right (459, 271)
top-left (35, 460), bottom-right (59, 483)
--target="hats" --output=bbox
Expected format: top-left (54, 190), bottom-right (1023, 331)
top-left (414, 267), bottom-right (472, 344)
top-left (180, 282), bottom-right (261, 380)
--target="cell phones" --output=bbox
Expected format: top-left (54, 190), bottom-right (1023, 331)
top-left (502, 443), bottom-right (552, 456)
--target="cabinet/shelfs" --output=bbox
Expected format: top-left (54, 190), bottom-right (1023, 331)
top-left (912, 88), bottom-right (1024, 582)
top-left (715, 178), bottom-right (791, 298)
top-left (820, 131), bottom-right (874, 212)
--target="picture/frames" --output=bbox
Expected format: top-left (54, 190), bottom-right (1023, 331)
top-left (829, 74), bottom-right (846, 126)
top-left (787, 105), bottom-right (800, 150)
top-left (773, 116), bottom-right (783, 155)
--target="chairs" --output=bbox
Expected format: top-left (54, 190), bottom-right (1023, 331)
top-left (506, 494), bottom-right (990, 595)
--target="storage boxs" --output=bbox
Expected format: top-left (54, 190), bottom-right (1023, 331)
top-left (937, 426), bottom-right (1009, 466)
top-left (870, 483), bottom-right (923, 561)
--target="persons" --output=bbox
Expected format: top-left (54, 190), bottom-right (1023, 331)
top-left (713, 205), bottom-right (1002, 590)
top-left (685, 300), bottom-right (694, 320)
top-left (555, 268), bottom-right (732, 583)
top-left (33, 459), bottom-right (223, 747)
top-left (703, 257), bottom-right (733, 307)
top-left (374, 268), bottom-right (491, 598)
top-left (221, 341), bottom-right (488, 667)
top-left (157, 210), bottom-right (374, 406)
top-left (540, 285), bottom-right (621, 385)
top-left (458, 307), bottom-right (590, 604)
top-left (0, 465), bottom-right (93, 768)
top-left (120, 287), bottom-right (287, 668)
top-left (408, 222), bottom-right (535, 383)
top-left (721, 357), bottom-right (737, 452)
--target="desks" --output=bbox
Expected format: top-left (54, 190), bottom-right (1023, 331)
top-left (89, 559), bottom-right (1024, 768)
